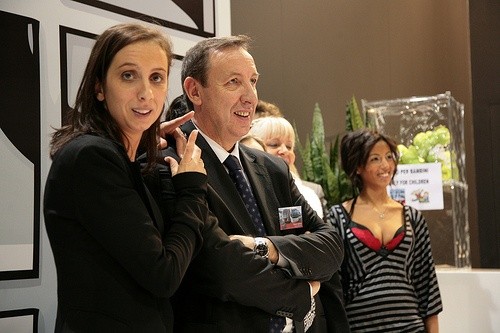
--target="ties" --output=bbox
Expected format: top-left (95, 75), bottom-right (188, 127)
top-left (223, 155), bottom-right (269, 238)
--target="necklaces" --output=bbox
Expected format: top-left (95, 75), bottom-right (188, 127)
top-left (371, 202), bottom-right (390, 218)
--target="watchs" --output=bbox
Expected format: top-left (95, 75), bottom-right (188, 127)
top-left (253, 236), bottom-right (270, 258)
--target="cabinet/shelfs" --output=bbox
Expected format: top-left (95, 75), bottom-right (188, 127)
top-left (361, 92), bottom-right (474, 271)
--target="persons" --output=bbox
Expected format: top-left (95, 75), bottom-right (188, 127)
top-left (326, 130), bottom-right (444, 333)
top-left (43, 24), bottom-right (207, 332)
top-left (138, 33), bottom-right (345, 332)
top-left (250, 115), bottom-right (323, 220)
